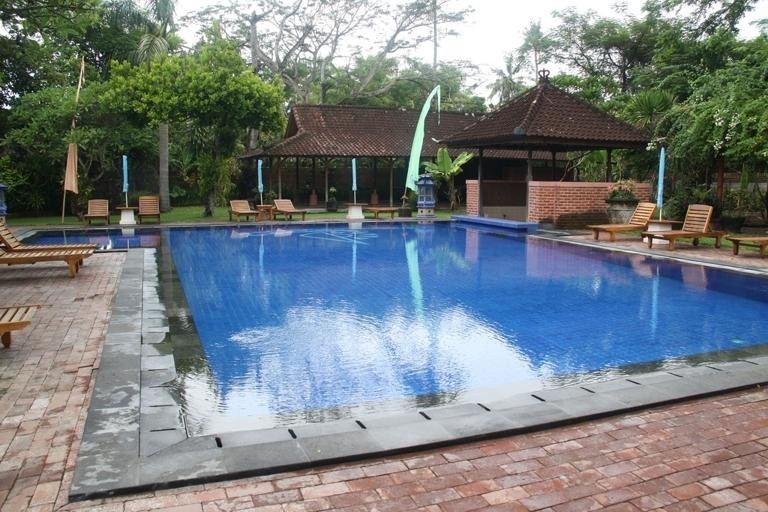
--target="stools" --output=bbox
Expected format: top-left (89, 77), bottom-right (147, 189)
top-left (366, 204), bottom-right (398, 218)
top-left (0, 295), bottom-right (41, 349)
top-left (726, 230), bottom-right (766, 255)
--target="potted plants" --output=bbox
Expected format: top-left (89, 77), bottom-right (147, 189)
top-left (605, 176), bottom-right (639, 225)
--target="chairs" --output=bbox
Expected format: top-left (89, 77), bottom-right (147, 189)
top-left (584, 195), bottom-right (724, 252)
top-left (84, 192), bottom-right (161, 223)
top-left (225, 190), bottom-right (308, 224)
top-left (2, 216), bottom-right (98, 277)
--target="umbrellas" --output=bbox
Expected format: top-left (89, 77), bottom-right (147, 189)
top-left (123, 155), bottom-right (129, 207)
top-left (352, 158), bottom-right (357, 203)
top-left (258, 159), bottom-right (264, 205)
top-left (657, 147), bottom-right (665, 221)
